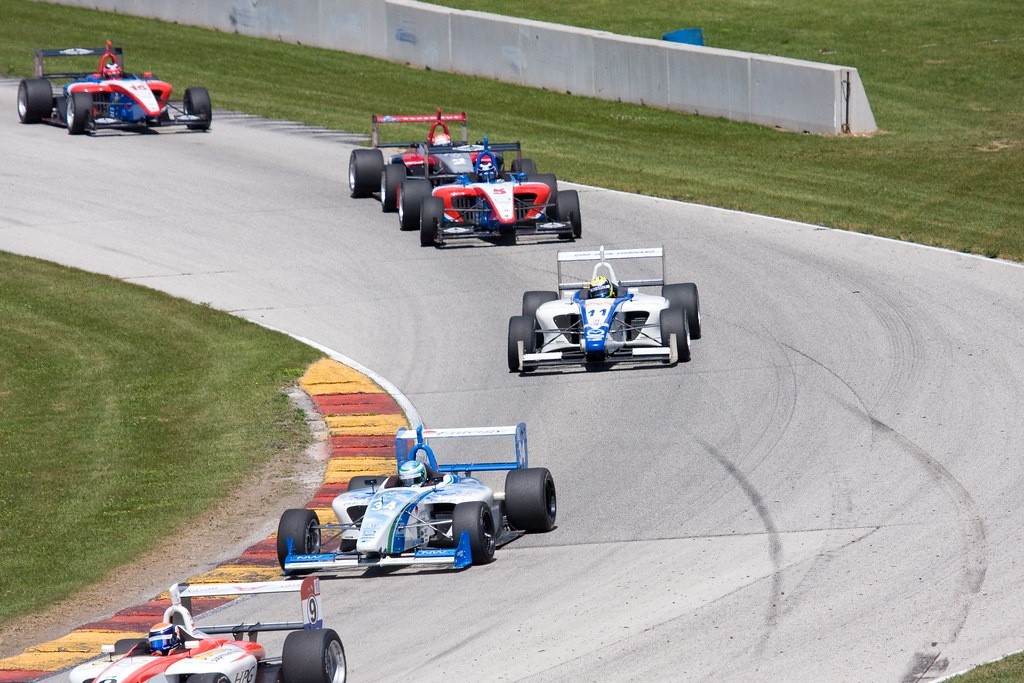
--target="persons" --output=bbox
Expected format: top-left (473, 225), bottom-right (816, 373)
top-left (103, 62), bottom-right (122, 81)
top-left (432, 133), bottom-right (452, 146)
top-left (149, 622), bottom-right (180, 655)
top-left (590, 276), bottom-right (613, 298)
top-left (397, 460), bottom-right (429, 488)
top-left (475, 161), bottom-right (498, 183)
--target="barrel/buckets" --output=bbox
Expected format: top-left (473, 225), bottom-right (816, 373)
top-left (662, 28), bottom-right (704, 46)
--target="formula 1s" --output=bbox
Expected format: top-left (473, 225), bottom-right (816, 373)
top-left (17, 41), bottom-right (213, 136)
top-left (507, 248), bottom-right (701, 375)
top-left (397, 139), bottom-right (582, 246)
top-left (70, 577), bottom-right (347, 683)
top-left (275, 423), bottom-right (554, 579)
top-left (350, 110), bottom-right (482, 199)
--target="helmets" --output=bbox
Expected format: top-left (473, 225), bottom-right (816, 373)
top-left (478, 162), bottom-right (498, 181)
top-left (590, 276), bottom-right (613, 298)
top-left (148, 622), bottom-right (180, 651)
top-left (102, 63), bottom-right (123, 82)
top-left (398, 460), bottom-right (428, 488)
top-left (432, 134), bottom-right (453, 148)
